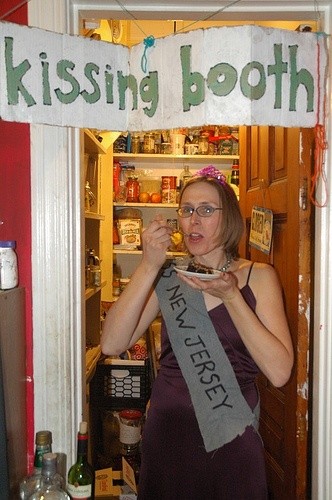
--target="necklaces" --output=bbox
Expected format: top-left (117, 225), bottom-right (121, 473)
top-left (219, 254), bottom-right (233, 271)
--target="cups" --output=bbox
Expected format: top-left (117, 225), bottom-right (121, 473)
top-left (170, 134), bottom-right (186, 155)
top-left (161, 175), bottom-right (177, 204)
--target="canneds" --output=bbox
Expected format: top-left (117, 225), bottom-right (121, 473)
top-left (198, 136), bottom-right (209, 155)
top-left (125, 177), bottom-right (139, 203)
top-left (117, 409), bottom-right (142, 455)
top-left (130, 133), bottom-right (173, 154)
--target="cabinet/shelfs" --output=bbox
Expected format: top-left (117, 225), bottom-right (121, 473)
top-left (98, 142), bottom-right (240, 304)
top-left (80, 127), bottom-right (107, 467)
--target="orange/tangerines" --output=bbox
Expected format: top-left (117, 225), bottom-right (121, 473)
top-left (138, 192), bottom-right (161, 203)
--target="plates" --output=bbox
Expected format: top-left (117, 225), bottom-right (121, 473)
top-left (172, 266), bottom-right (222, 280)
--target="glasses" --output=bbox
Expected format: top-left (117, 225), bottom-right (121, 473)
top-left (176, 206), bottom-right (224, 218)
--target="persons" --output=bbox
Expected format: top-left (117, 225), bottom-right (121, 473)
top-left (100, 175), bottom-right (293, 500)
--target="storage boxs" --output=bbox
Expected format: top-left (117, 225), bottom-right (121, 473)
top-left (115, 218), bottom-right (143, 248)
top-left (90, 357), bottom-right (152, 409)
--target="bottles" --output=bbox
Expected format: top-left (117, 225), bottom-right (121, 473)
top-left (111, 410), bottom-right (143, 486)
top-left (230, 160), bottom-right (239, 187)
top-left (65, 421), bottom-right (95, 500)
top-left (18, 430), bottom-right (65, 500)
top-left (179, 165), bottom-right (192, 190)
top-left (29, 452), bottom-right (71, 500)
top-left (0, 240), bottom-right (19, 290)
top-left (125, 177), bottom-right (140, 203)
top-left (197, 136), bottom-right (209, 155)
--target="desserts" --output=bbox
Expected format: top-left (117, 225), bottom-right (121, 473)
top-left (187, 262), bottom-right (213, 274)
